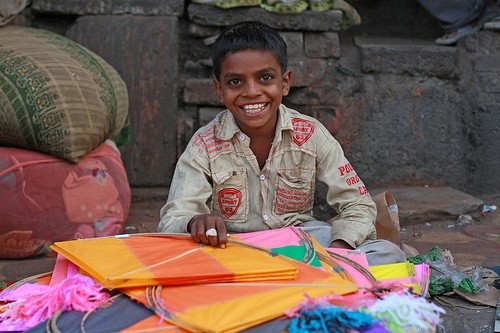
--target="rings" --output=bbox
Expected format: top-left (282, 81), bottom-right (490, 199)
top-left (206, 229), bottom-right (217, 238)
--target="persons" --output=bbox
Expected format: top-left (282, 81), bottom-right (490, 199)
top-left (156, 21), bottom-right (378, 261)
